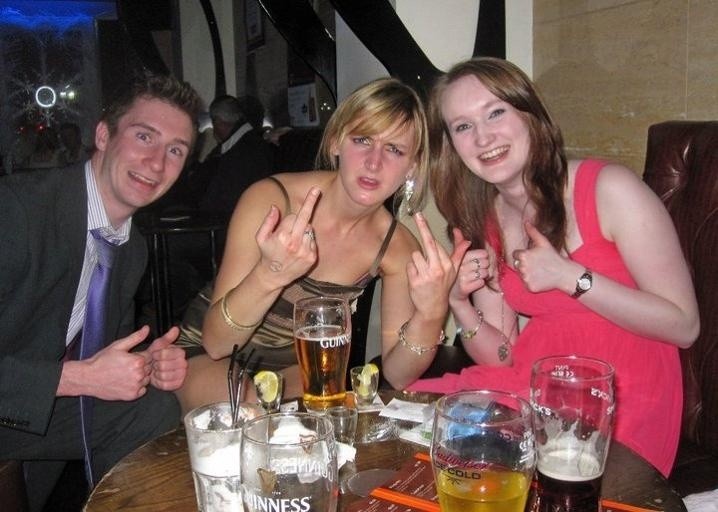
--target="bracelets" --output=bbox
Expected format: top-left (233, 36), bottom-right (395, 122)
top-left (222, 288), bottom-right (265, 331)
top-left (460, 307), bottom-right (482, 338)
top-left (398, 319), bottom-right (446, 355)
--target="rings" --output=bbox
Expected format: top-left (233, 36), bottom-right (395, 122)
top-left (473, 259), bottom-right (481, 270)
top-left (302, 230), bottom-right (314, 240)
top-left (474, 271), bottom-right (480, 280)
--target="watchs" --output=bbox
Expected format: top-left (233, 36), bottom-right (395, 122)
top-left (571, 267), bottom-right (593, 300)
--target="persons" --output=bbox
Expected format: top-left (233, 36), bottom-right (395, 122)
top-left (190, 94), bottom-right (292, 172)
top-left (178, 94), bottom-right (290, 222)
top-left (1, 70), bottom-right (209, 509)
top-left (167, 76), bottom-right (473, 431)
top-left (403, 59), bottom-right (700, 478)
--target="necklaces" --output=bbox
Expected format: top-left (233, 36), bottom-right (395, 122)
top-left (498, 168), bottom-right (551, 360)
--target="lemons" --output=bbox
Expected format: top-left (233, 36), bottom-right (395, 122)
top-left (358, 364), bottom-right (378, 397)
top-left (253, 370), bottom-right (278, 403)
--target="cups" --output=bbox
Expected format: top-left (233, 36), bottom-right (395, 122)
top-left (325, 406), bottom-right (358, 447)
top-left (292, 296), bottom-right (352, 415)
top-left (528, 354), bottom-right (615, 512)
top-left (351, 367), bottom-right (379, 405)
top-left (239, 412), bottom-right (338, 511)
top-left (184, 400), bottom-right (270, 512)
top-left (253, 372), bottom-right (282, 414)
top-left (429, 389), bottom-right (536, 512)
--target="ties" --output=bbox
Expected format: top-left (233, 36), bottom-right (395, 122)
top-left (77, 227), bottom-right (118, 491)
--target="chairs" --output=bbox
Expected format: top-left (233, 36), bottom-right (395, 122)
top-left (139, 221), bottom-right (229, 340)
top-left (640, 121), bottom-right (716, 495)
